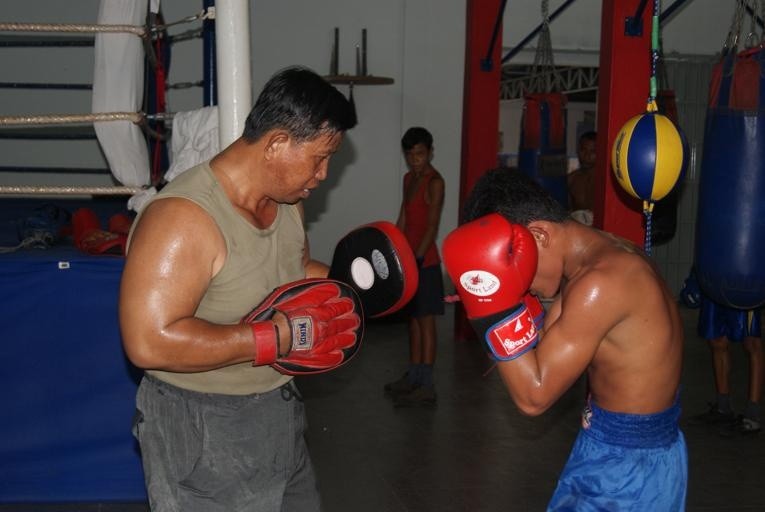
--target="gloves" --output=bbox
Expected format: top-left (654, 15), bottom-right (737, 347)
top-left (443, 212), bottom-right (547, 361)
top-left (326, 221), bottom-right (419, 319)
top-left (18, 213), bottom-right (53, 250)
top-left (71, 208), bottom-right (136, 255)
top-left (240, 278), bottom-right (365, 376)
top-left (519, 289), bottom-right (546, 332)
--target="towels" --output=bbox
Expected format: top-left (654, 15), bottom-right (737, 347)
top-left (164, 105), bottom-right (218, 182)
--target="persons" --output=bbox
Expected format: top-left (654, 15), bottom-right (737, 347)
top-left (679, 240), bottom-right (765, 437)
top-left (388, 124), bottom-right (447, 405)
top-left (443, 167), bottom-right (693, 511)
top-left (118, 67), bottom-right (420, 510)
top-left (566, 130), bottom-right (604, 227)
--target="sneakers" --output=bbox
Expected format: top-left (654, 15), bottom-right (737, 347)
top-left (385, 372), bottom-right (437, 405)
top-left (683, 404), bottom-right (738, 436)
top-left (716, 410), bottom-right (765, 438)
